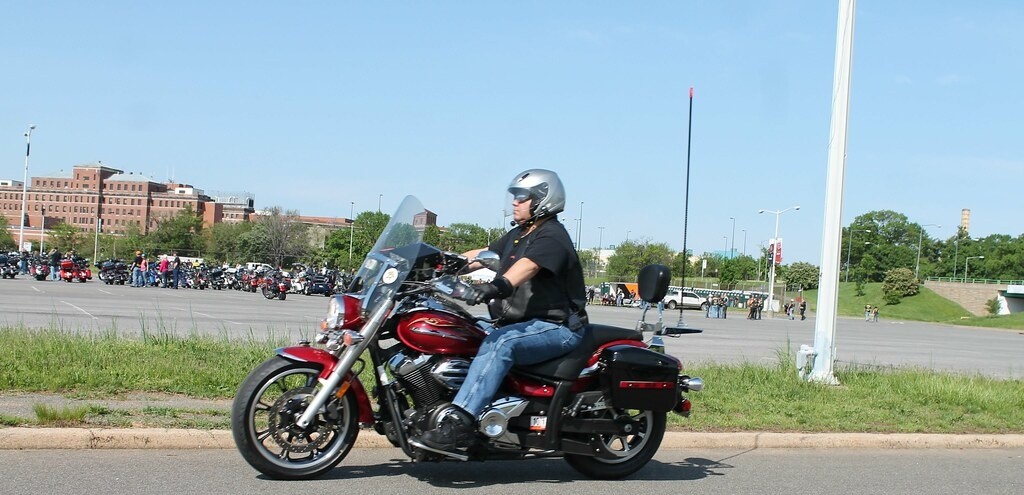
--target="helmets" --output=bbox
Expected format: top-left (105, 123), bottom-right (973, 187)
top-left (508, 169), bottom-right (565, 218)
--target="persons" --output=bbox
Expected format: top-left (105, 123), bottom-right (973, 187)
top-left (705, 292), bottom-right (730, 320)
top-left (172, 251), bottom-right (181, 289)
top-left (421, 169), bottom-right (591, 452)
top-left (789, 299), bottom-right (795, 320)
top-left (160, 253), bottom-right (172, 288)
top-left (49, 246), bottom-right (61, 281)
top-left (864, 303), bottom-right (879, 322)
top-left (800, 299), bottom-right (807, 320)
top-left (288, 264), bottom-right (320, 279)
top-left (130, 251), bottom-right (149, 287)
top-left (236, 263), bottom-right (241, 269)
top-left (747, 295), bottom-right (764, 320)
top-left (587, 288), bottom-right (594, 302)
top-left (186, 259), bottom-right (200, 268)
top-left (20, 248), bottom-right (31, 274)
top-left (604, 290), bottom-right (625, 307)
top-left (333, 267), bottom-right (355, 282)
top-left (213, 261), bottom-right (229, 269)
top-left (629, 289), bottom-right (636, 304)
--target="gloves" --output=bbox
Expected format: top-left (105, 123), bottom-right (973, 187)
top-left (461, 276), bottom-right (514, 305)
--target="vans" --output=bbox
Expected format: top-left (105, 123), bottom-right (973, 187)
top-left (245, 262), bottom-right (292, 279)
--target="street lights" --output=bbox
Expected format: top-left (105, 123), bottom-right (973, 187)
top-left (729, 216), bottom-right (735, 259)
top-left (349, 200), bottom-right (356, 258)
top-left (759, 206), bottom-right (801, 318)
top-left (377, 194), bottom-right (383, 213)
top-left (742, 229), bottom-right (746, 257)
top-left (627, 230), bottom-right (631, 241)
top-left (845, 229), bottom-right (872, 282)
top-left (598, 226), bottom-right (605, 252)
top-left (964, 255), bottom-right (985, 283)
top-left (916, 223), bottom-right (942, 279)
top-left (723, 236), bottom-right (727, 257)
top-left (574, 219), bottom-right (581, 251)
top-left (18, 123), bottom-right (36, 252)
top-left (578, 201), bottom-right (585, 251)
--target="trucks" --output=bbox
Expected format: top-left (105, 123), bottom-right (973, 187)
top-left (600, 281), bottom-right (641, 305)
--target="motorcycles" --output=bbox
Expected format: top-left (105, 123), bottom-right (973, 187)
top-left (0, 249), bottom-right (92, 284)
top-left (230, 193), bottom-right (705, 482)
top-left (94, 255), bottom-right (288, 301)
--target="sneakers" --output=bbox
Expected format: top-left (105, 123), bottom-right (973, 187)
top-left (407, 411), bottom-right (472, 461)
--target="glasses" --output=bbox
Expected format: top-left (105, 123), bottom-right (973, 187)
top-left (513, 194), bottom-right (534, 202)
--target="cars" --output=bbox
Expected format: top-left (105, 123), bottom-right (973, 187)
top-left (304, 276), bottom-right (331, 297)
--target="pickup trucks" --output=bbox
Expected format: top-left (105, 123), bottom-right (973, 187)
top-left (663, 289), bottom-right (707, 312)
